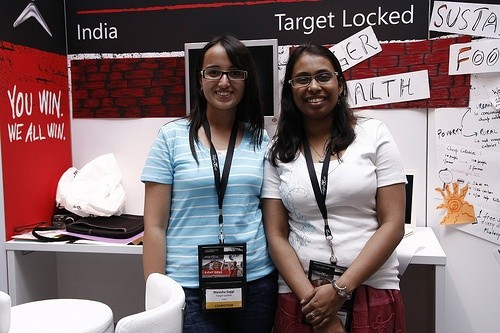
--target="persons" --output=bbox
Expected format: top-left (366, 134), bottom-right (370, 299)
top-left (262, 43), bottom-right (409, 333)
top-left (139, 36), bottom-right (281, 333)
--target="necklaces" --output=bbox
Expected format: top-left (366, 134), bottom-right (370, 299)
top-left (308, 129), bottom-right (330, 162)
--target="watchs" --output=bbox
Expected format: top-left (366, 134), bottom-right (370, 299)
top-left (332, 281), bottom-right (352, 302)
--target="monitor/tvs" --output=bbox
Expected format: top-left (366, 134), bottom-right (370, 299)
top-left (184, 39), bottom-right (279, 125)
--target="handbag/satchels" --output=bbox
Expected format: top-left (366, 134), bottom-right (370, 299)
top-left (66, 215), bottom-right (144, 239)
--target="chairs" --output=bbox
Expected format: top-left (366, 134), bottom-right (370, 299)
top-left (115, 273), bottom-right (185, 333)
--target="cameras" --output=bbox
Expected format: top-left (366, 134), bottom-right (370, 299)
top-left (52, 214), bottom-right (78, 229)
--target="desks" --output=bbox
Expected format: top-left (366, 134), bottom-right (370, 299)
top-left (6, 299), bottom-right (114, 333)
top-left (4, 228), bottom-right (447, 333)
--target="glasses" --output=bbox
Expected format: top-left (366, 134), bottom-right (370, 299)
top-left (199, 68), bottom-right (248, 81)
top-left (287, 72), bottom-right (340, 89)
